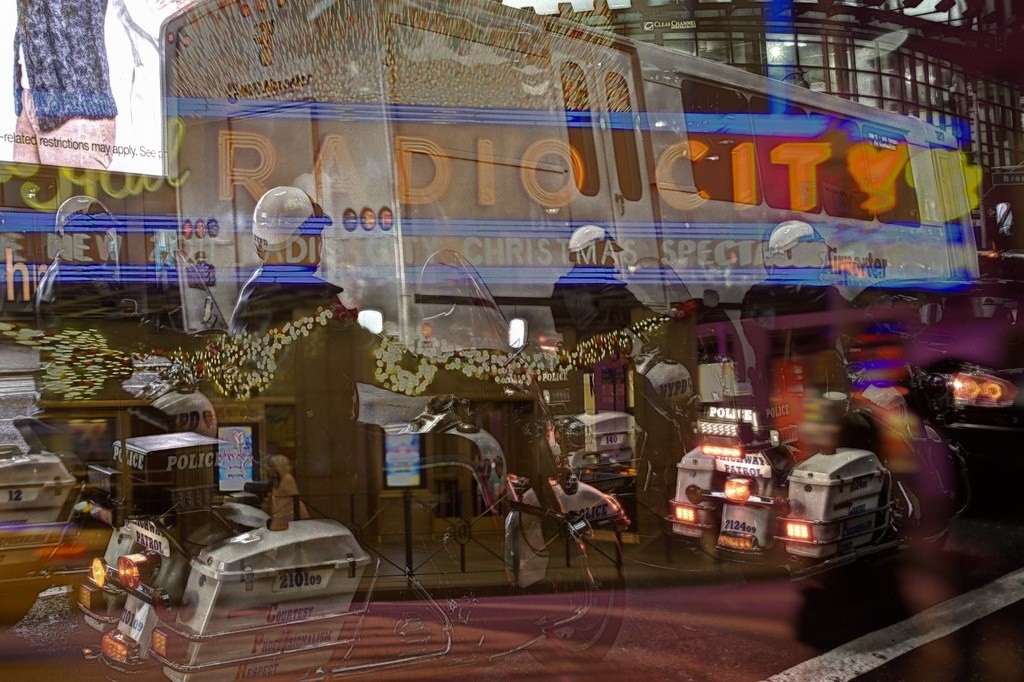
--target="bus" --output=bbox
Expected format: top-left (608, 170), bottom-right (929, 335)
top-left (153, 0), bottom-right (980, 337)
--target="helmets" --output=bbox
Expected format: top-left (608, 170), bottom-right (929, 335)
top-left (768, 221), bottom-right (826, 266)
top-left (253, 183), bottom-right (325, 250)
top-left (569, 223), bottom-right (623, 259)
top-left (57, 197), bottom-right (113, 236)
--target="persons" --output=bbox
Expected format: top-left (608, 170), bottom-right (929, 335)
top-left (11, 0), bottom-right (118, 171)
top-left (36, 187), bottom-right (916, 532)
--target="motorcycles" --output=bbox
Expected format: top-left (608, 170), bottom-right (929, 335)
top-left (0, 247), bottom-right (272, 632)
top-left (517, 251), bottom-right (749, 536)
top-left (665, 256), bottom-right (977, 591)
top-left (52, 247), bottom-right (634, 681)
